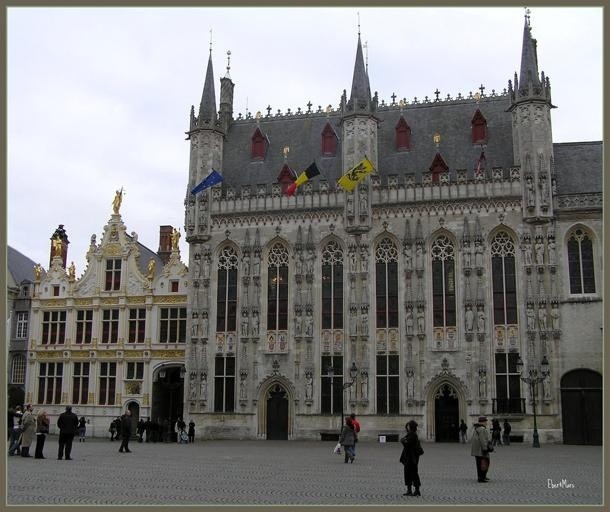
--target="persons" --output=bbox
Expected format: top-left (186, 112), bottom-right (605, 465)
top-left (112, 190), bottom-right (122, 214)
top-left (471, 416), bottom-right (493, 483)
top-left (34, 263), bottom-right (42, 280)
top-left (348, 413), bottom-right (360, 456)
top-left (376, 329), bottom-right (399, 352)
top-left (339, 416), bottom-right (355, 464)
top-left (399, 420), bottom-right (424, 497)
top-left (54, 235), bottom-right (63, 256)
top-left (69, 261), bottom-right (75, 282)
top-left (170, 227), bottom-right (181, 254)
top-left (460, 419), bottom-right (468, 442)
top-left (7, 402), bottom-right (87, 460)
top-left (189, 174), bottom-right (563, 406)
top-left (321, 329), bottom-right (344, 354)
top-left (148, 257), bottom-right (156, 276)
top-left (117, 409), bottom-right (134, 453)
top-left (111, 415), bottom-right (195, 443)
top-left (491, 418), bottom-right (513, 447)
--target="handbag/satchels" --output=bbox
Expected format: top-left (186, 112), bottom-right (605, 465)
top-left (418, 440), bottom-right (425, 456)
top-left (488, 440), bottom-right (494, 452)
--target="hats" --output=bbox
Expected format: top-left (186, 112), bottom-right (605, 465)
top-left (478, 416), bottom-right (488, 422)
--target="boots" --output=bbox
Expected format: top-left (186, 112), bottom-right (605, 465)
top-left (403, 485), bottom-right (421, 497)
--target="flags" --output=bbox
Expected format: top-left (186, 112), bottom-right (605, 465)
top-left (337, 159), bottom-right (373, 193)
top-left (190, 171), bottom-right (224, 195)
top-left (284, 162), bottom-right (320, 197)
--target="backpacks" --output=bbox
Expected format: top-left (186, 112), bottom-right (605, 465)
top-left (355, 423), bottom-right (361, 432)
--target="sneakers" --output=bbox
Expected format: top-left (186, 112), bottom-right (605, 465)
top-left (478, 478), bottom-right (490, 483)
